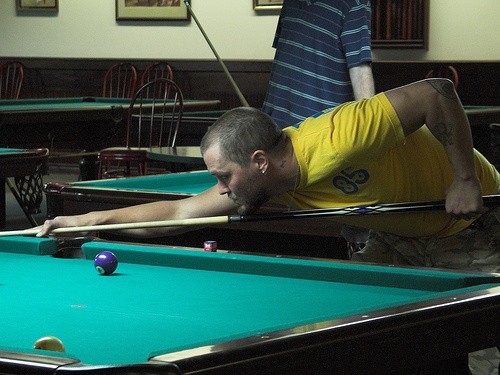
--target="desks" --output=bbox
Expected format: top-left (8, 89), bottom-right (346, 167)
top-left (0, 233), bottom-right (500, 375)
top-left (0, 148), bottom-right (49, 228)
top-left (462, 104), bottom-right (500, 126)
top-left (0, 95), bottom-right (220, 179)
top-left (145, 146), bottom-right (204, 172)
top-left (42, 169), bottom-right (218, 219)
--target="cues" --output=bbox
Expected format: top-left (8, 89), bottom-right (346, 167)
top-left (0, 192), bottom-right (500, 237)
top-left (184, 0), bottom-right (251, 108)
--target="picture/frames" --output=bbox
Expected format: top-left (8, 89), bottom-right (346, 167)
top-left (115, 0), bottom-right (191, 23)
top-left (16, 0), bottom-right (58, 12)
top-left (252, 0), bottom-right (283, 11)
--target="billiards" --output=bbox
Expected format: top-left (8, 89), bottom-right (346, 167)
top-left (32, 336), bottom-right (65, 352)
top-left (94, 251), bottom-right (118, 276)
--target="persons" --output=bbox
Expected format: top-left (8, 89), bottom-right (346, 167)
top-left (261, 0), bottom-right (375, 131)
top-left (26, 78), bottom-right (500, 375)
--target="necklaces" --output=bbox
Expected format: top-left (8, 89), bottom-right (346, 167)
top-left (279, 165), bottom-right (301, 212)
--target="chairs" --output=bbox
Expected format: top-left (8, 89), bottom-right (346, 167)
top-left (0, 59), bottom-right (183, 228)
